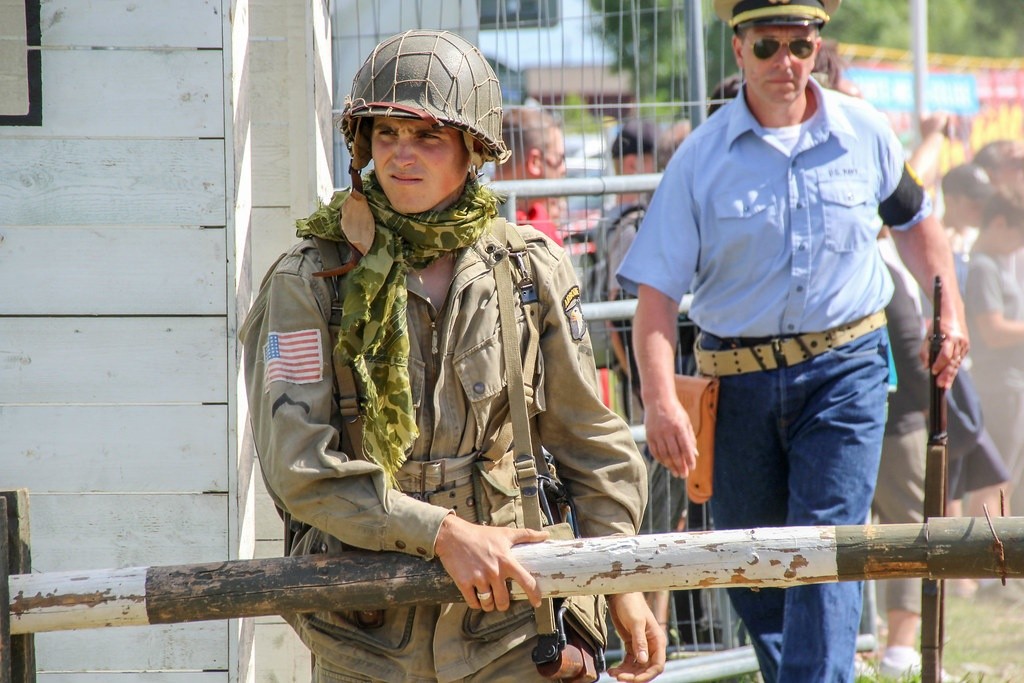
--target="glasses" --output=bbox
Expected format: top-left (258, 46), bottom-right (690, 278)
top-left (742, 35), bottom-right (820, 60)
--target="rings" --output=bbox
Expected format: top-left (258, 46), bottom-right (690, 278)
top-left (476, 592), bottom-right (492, 600)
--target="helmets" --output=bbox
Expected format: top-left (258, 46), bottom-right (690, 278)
top-left (342, 28), bottom-right (508, 166)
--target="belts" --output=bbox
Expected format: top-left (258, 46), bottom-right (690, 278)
top-left (693, 309), bottom-right (887, 378)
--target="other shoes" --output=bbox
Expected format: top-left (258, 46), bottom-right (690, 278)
top-left (882, 656), bottom-right (952, 682)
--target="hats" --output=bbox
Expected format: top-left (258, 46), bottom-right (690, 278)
top-left (714, 0), bottom-right (842, 35)
top-left (599, 119), bottom-right (661, 158)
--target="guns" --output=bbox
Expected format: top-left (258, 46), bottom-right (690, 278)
top-left (530, 449), bottom-right (633, 683)
top-left (918, 280), bottom-right (951, 683)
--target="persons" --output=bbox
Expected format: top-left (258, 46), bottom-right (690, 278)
top-left (617, 1), bottom-right (972, 683)
top-left (239, 30), bottom-right (667, 683)
top-left (488, 97), bottom-right (1024, 683)
